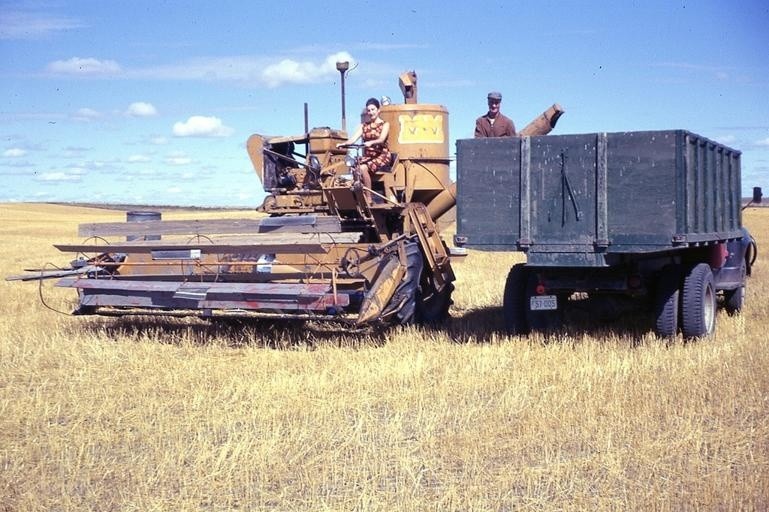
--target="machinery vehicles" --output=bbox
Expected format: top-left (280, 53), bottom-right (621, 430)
top-left (5, 57), bottom-right (565, 339)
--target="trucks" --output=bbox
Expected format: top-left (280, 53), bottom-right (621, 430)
top-left (454, 129), bottom-right (762, 341)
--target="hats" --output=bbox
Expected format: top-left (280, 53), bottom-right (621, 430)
top-left (488, 91), bottom-right (503, 102)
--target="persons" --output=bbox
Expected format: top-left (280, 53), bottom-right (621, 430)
top-left (473, 91), bottom-right (516, 137)
top-left (335, 97), bottom-right (392, 206)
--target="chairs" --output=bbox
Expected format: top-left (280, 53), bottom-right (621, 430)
top-left (372, 153), bottom-right (398, 200)
